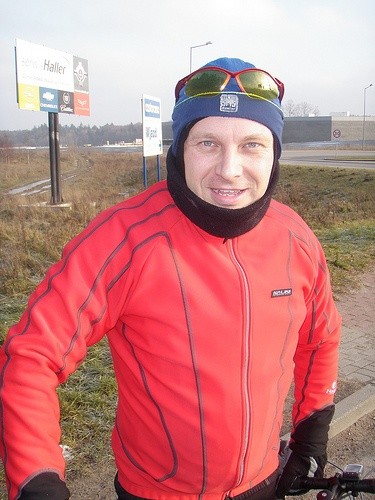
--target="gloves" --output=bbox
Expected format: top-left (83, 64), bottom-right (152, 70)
top-left (275, 452), bottom-right (327, 496)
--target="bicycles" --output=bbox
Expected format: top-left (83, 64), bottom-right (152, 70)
top-left (290, 464), bottom-right (375, 500)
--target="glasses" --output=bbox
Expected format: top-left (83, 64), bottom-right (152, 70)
top-left (175, 66), bottom-right (284, 102)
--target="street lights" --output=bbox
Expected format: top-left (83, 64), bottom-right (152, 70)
top-left (361, 83), bottom-right (373, 149)
top-left (190, 41), bottom-right (212, 74)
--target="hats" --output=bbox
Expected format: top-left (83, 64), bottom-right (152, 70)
top-left (172, 57), bottom-right (284, 159)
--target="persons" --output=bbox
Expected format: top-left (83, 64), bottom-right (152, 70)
top-left (0, 57), bottom-right (342, 500)
